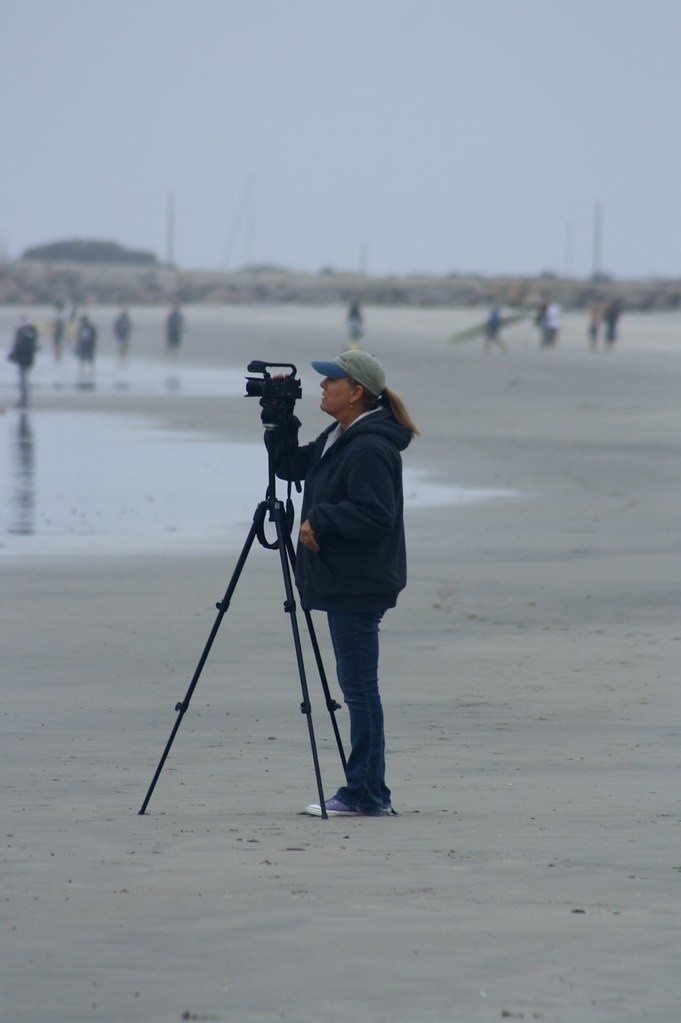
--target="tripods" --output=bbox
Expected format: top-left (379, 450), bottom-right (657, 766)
top-left (138, 400), bottom-right (357, 818)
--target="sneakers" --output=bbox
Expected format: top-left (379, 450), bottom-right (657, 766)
top-left (305, 798), bottom-right (384, 816)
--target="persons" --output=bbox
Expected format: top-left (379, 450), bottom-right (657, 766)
top-left (345, 297), bottom-right (364, 351)
top-left (165, 301), bottom-right (187, 358)
top-left (6, 318), bottom-right (41, 409)
top-left (260, 347), bottom-right (422, 820)
top-left (50, 302), bottom-right (70, 363)
top-left (110, 310), bottom-right (133, 363)
top-left (533, 297), bottom-right (621, 346)
top-left (482, 304), bottom-right (506, 356)
top-left (70, 315), bottom-right (98, 381)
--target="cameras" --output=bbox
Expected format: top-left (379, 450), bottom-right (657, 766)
top-left (239, 359), bottom-right (305, 404)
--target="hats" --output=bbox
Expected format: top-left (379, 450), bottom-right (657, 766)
top-left (311, 350), bottom-right (385, 397)
